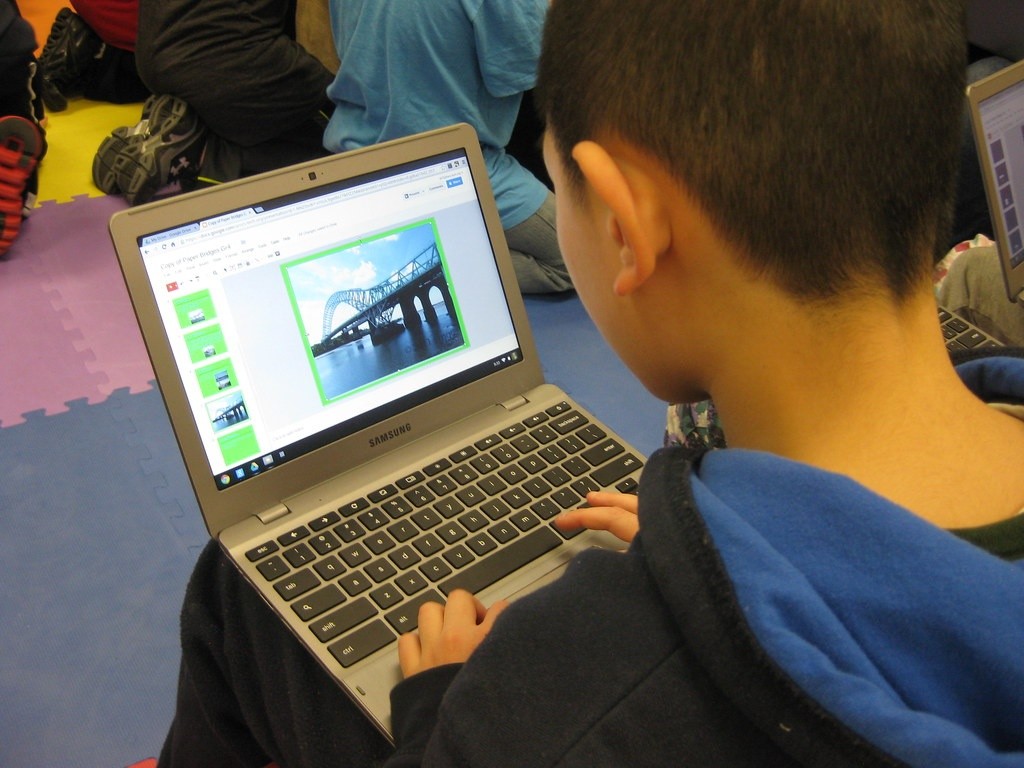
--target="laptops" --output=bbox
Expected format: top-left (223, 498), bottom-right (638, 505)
top-left (937, 305), bottom-right (1006, 353)
top-left (962, 58), bottom-right (1024, 310)
top-left (107, 122), bottom-right (646, 747)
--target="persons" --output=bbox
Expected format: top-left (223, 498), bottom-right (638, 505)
top-left (152, 0), bottom-right (1024, 768)
top-left (0, 0), bottom-right (584, 297)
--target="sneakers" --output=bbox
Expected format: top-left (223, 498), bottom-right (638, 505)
top-left (31, 8), bottom-right (103, 112)
top-left (0, 116), bottom-right (41, 257)
top-left (93, 93), bottom-right (209, 206)
